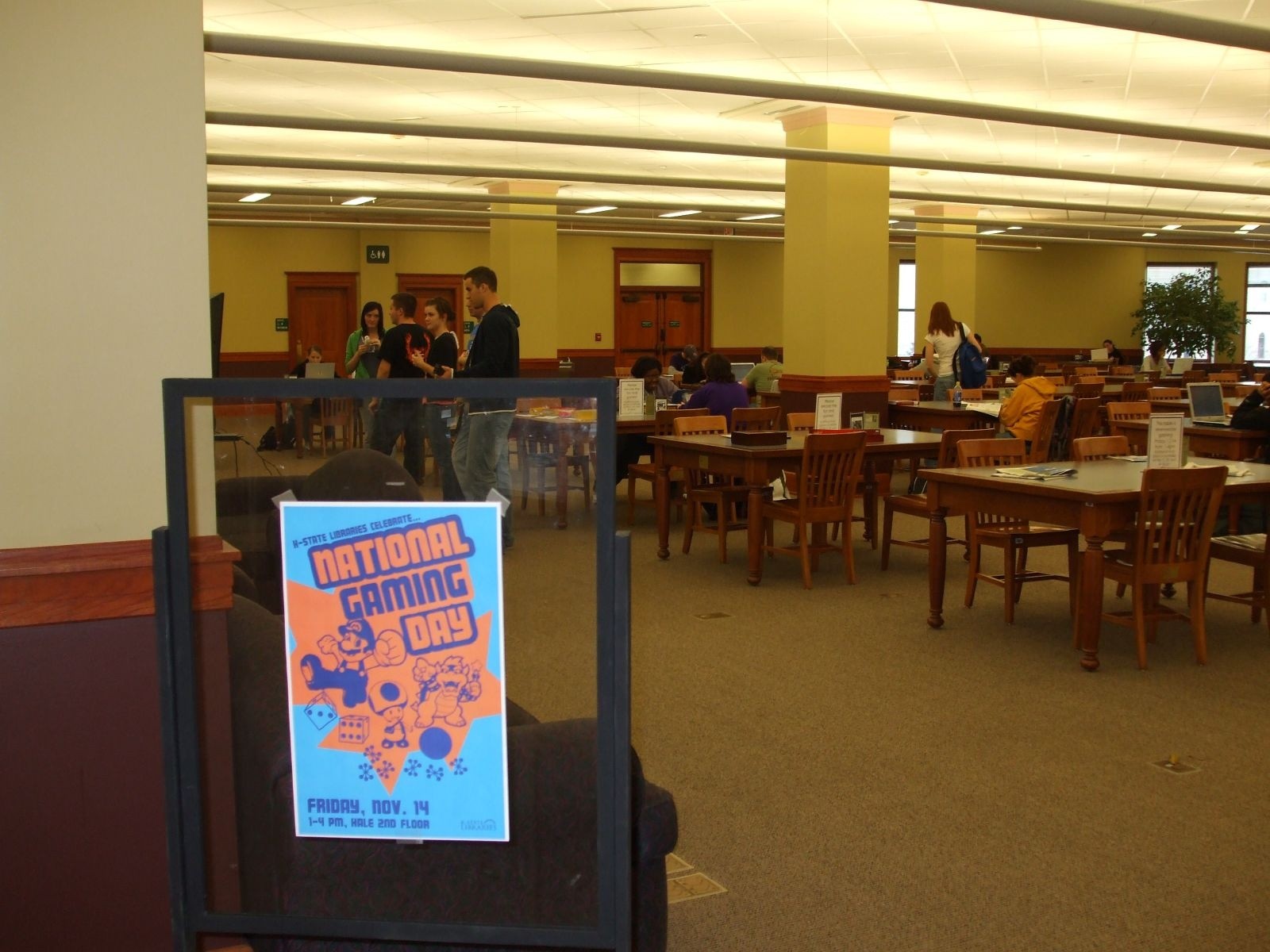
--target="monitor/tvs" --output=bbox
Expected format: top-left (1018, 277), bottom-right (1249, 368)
top-left (730, 363), bottom-right (755, 384)
top-left (304, 363), bottom-right (336, 378)
top-left (1090, 348), bottom-right (1110, 360)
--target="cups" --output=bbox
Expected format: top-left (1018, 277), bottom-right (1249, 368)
top-left (364, 338), bottom-right (376, 353)
top-left (646, 393), bottom-right (654, 416)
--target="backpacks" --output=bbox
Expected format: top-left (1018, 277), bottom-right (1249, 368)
top-left (952, 321), bottom-right (987, 388)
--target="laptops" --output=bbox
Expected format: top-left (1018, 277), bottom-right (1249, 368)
top-left (1166, 358), bottom-right (1194, 376)
top-left (1186, 381), bottom-right (1231, 426)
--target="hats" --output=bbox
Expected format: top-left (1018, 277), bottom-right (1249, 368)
top-left (684, 344), bottom-right (699, 360)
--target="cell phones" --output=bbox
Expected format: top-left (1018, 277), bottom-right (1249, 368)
top-left (433, 363), bottom-right (445, 376)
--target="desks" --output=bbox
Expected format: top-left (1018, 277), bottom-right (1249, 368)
top-left (889, 379), bottom-right (935, 390)
top-left (646, 427), bottom-right (944, 588)
top-left (883, 400), bottom-right (1005, 434)
top-left (917, 454), bottom-right (1270, 673)
top-left (978, 384), bottom-right (1124, 406)
top-left (1138, 398), bottom-right (1192, 419)
top-left (1105, 415), bottom-right (1270, 462)
top-left (1098, 376), bottom-right (1184, 389)
top-left (1216, 382), bottom-right (1261, 398)
top-left (274, 397), bottom-right (364, 460)
top-left (513, 408), bottom-right (655, 527)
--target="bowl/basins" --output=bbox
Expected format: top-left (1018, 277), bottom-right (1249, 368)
top-left (573, 409), bottom-right (597, 420)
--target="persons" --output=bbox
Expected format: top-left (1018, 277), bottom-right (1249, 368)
top-left (1103, 340), bottom-right (1124, 366)
top-left (431, 265), bottom-right (520, 548)
top-left (593, 345), bottom-right (784, 522)
top-left (996, 354), bottom-right (1057, 454)
top-left (286, 345), bottom-right (340, 452)
top-left (346, 292), bottom-right (485, 501)
top-left (917, 334), bottom-right (990, 369)
top-left (1141, 340), bottom-right (1172, 375)
top-left (1231, 372), bottom-right (1270, 464)
top-left (924, 301), bottom-right (982, 467)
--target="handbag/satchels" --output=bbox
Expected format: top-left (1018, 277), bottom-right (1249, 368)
top-left (258, 426), bottom-right (276, 453)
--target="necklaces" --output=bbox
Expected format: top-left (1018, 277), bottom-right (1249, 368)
top-left (645, 390), bottom-right (655, 399)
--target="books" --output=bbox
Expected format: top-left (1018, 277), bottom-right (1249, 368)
top-left (1185, 457), bottom-right (1254, 478)
top-left (990, 465), bottom-right (1078, 481)
top-left (516, 413), bottom-right (559, 419)
top-left (965, 402), bottom-right (1003, 418)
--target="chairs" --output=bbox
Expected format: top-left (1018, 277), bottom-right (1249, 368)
top-left (275, 322), bottom-right (1270, 671)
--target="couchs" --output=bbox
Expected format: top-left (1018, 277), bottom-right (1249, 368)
top-left (215, 449), bottom-right (681, 952)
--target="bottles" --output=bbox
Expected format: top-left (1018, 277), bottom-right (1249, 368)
top-left (953, 381), bottom-right (962, 407)
top-left (1079, 351), bottom-right (1082, 362)
